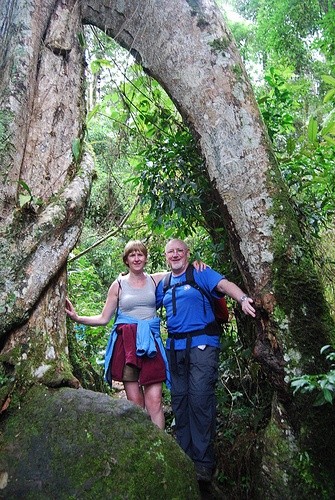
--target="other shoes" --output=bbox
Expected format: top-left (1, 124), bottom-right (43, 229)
top-left (193, 460), bottom-right (217, 483)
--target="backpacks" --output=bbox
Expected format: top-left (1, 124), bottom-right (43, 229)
top-left (162, 263), bottom-right (230, 324)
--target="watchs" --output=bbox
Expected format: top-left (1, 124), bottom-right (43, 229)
top-left (239, 293), bottom-right (249, 305)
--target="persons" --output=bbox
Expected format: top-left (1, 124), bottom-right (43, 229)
top-left (65, 240), bottom-right (211, 433)
top-left (153, 239), bottom-right (257, 484)
